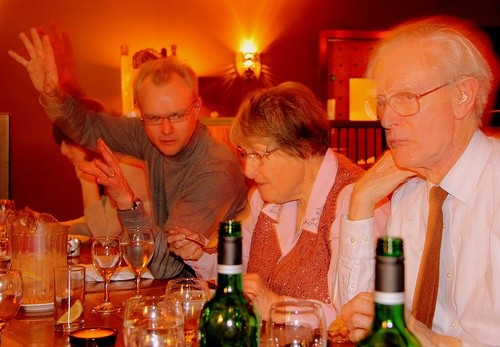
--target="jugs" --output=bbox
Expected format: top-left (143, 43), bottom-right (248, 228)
top-left (5, 210), bottom-right (70, 308)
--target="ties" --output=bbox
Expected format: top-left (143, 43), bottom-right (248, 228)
top-left (411, 187), bottom-right (449, 330)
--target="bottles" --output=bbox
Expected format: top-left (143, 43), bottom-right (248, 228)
top-left (353, 239), bottom-right (423, 347)
top-left (195, 219), bottom-right (259, 347)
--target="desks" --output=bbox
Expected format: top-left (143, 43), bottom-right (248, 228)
top-left (0, 243), bottom-right (356, 347)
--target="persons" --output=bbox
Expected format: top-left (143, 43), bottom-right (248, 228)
top-left (330, 24), bottom-right (500, 347)
top-left (7, 28), bottom-right (248, 281)
top-left (55, 97), bottom-right (153, 249)
top-left (167, 82), bottom-right (391, 331)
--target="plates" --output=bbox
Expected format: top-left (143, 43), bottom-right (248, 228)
top-left (67, 234), bottom-right (89, 243)
top-left (19, 288), bottom-right (75, 311)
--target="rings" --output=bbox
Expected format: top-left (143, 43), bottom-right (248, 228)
top-left (108, 171), bottom-right (116, 177)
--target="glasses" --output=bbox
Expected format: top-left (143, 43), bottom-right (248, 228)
top-left (237, 144), bottom-right (283, 168)
top-left (140, 99), bottom-right (197, 126)
top-left (364, 79), bottom-right (457, 121)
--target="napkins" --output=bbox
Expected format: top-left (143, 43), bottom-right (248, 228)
top-left (73, 260), bottom-right (154, 279)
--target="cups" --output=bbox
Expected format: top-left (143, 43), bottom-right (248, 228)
top-left (122, 294), bottom-right (186, 347)
top-left (53, 266), bottom-right (86, 335)
top-left (0, 269), bottom-right (23, 340)
top-left (0, 198), bottom-right (17, 261)
top-left (265, 301), bottom-right (328, 347)
top-left (67, 238), bottom-right (80, 257)
top-left (68, 328), bottom-right (117, 347)
top-left (164, 278), bottom-right (209, 347)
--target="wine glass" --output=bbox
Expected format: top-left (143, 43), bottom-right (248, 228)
top-left (119, 227), bottom-right (156, 309)
top-left (89, 235), bottom-right (122, 313)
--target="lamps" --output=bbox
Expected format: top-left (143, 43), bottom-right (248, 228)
top-left (242, 52), bottom-right (256, 81)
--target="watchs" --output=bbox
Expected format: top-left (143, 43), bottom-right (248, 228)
top-left (116, 197), bottom-right (141, 211)
